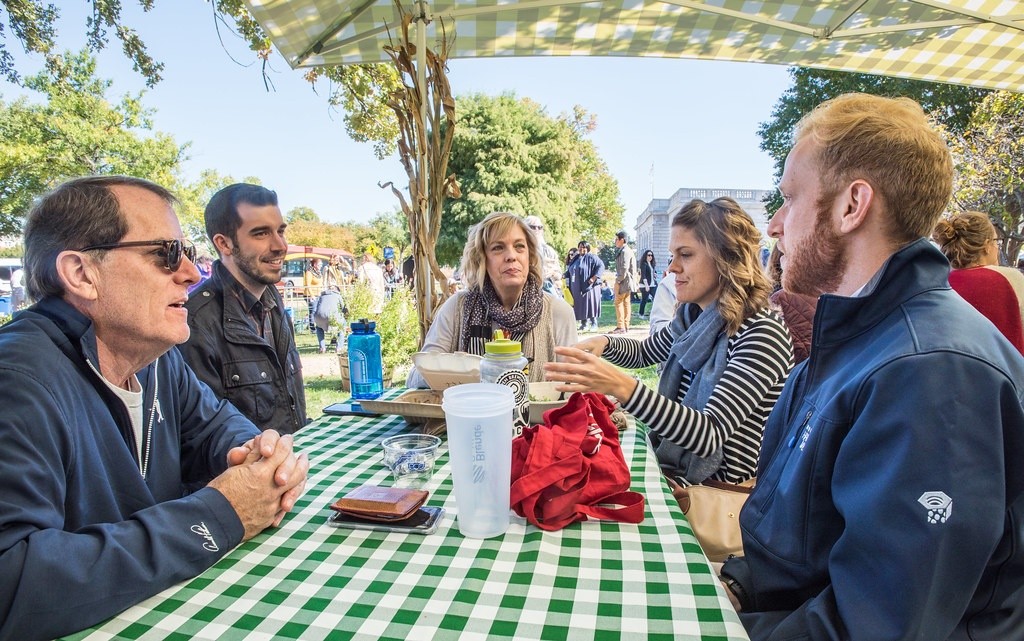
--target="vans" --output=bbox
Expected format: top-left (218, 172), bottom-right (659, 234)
top-left (0, 259), bottom-right (23, 291)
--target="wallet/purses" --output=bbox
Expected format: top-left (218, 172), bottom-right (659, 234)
top-left (330, 484), bottom-right (429, 521)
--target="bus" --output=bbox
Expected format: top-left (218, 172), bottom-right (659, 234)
top-left (273, 246), bottom-right (354, 298)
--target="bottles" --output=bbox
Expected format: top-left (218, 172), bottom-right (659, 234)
top-left (348, 318), bottom-right (383, 400)
top-left (479, 329), bottom-right (530, 442)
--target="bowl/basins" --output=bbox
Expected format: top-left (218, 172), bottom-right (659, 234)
top-left (359, 387), bottom-right (447, 423)
top-left (526, 381), bottom-right (576, 426)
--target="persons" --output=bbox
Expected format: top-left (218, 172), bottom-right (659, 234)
top-left (10, 256), bottom-right (28, 311)
top-left (173, 183), bottom-right (314, 438)
top-left (303, 253), bottom-right (416, 353)
top-left (760, 211), bottom-right (1024, 366)
top-left (455, 214), bottom-right (681, 377)
top-left (543, 196), bottom-right (795, 486)
top-left (0, 166), bottom-right (310, 641)
top-left (406, 212), bottom-right (579, 387)
top-left (713, 91), bottom-right (1024, 641)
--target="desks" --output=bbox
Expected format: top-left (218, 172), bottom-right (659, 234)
top-left (55, 387), bottom-right (753, 641)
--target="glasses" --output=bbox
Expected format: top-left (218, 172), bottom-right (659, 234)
top-left (992, 237), bottom-right (1004, 244)
top-left (647, 254), bottom-right (652, 256)
top-left (78, 240), bottom-right (196, 273)
top-left (568, 254), bottom-right (575, 256)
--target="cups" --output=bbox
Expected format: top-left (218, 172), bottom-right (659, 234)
top-left (440, 383), bottom-right (516, 539)
top-left (381, 433), bottom-right (441, 500)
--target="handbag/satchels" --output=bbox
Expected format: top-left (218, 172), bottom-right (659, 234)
top-left (663, 475), bottom-right (759, 562)
top-left (510, 392), bottom-right (643, 531)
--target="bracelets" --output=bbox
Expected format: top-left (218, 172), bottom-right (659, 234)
top-left (717, 574), bottom-right (752, 613)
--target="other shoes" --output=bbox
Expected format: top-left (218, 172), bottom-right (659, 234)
top-left (609, 328), bottom-right (626, 334)
top-left (577, 325), bottom-right (587, 331)
top-left (589, 324), bottom-right (598, 331)
top-left (639, 315), bottom-right (648, 319)
